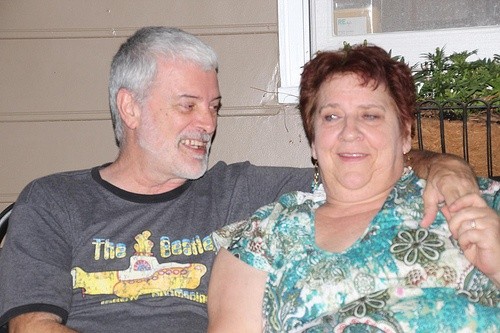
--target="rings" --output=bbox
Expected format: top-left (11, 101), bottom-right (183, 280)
top-left (471, 219), bottom-right (477, 230)
top-left (438, 200), bottom-right (447, 208)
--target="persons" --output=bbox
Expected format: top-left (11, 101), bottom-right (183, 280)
top-left (0, 25), bottom-right (479, 333)
top-left (204, 38), bottom-right (500, 333)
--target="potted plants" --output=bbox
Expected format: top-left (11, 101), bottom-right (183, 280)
top-left (409, 43), bottom-right (500, 178)
